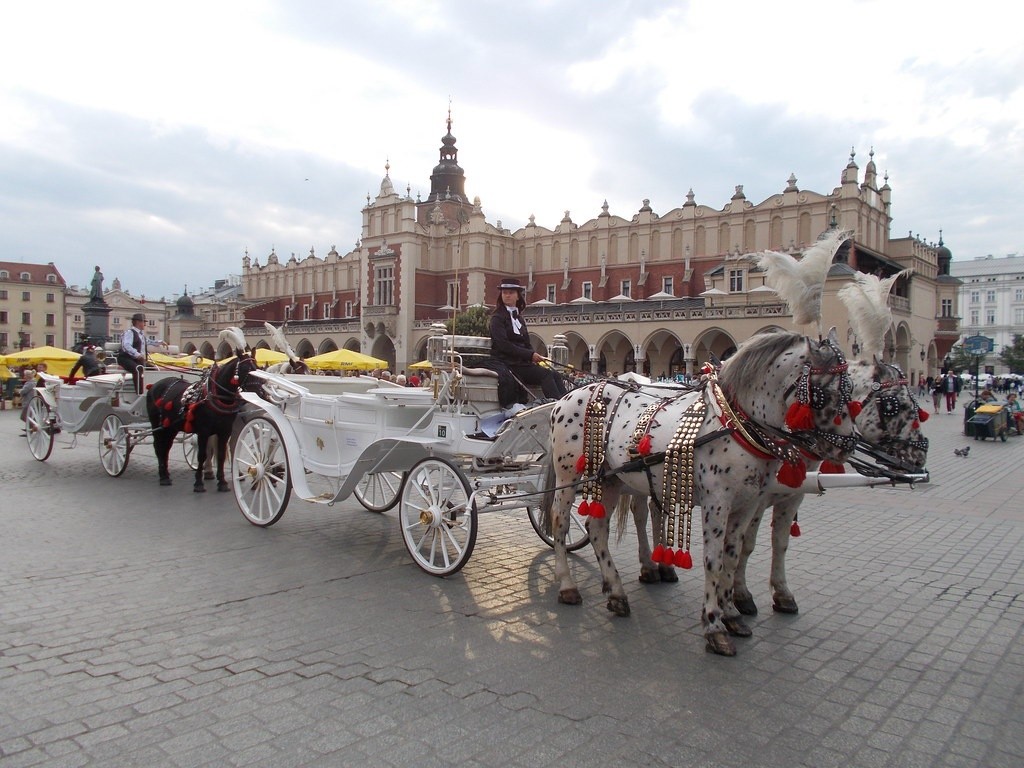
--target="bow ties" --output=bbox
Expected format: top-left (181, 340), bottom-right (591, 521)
top-left (512, 310), bottom-right (518, 320)
top-left (140, 331), bottom-right (144, 335)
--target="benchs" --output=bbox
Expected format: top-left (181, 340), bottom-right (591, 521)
top-left (281, 374), bottom-right (378, 396)
top-left (441, 334), bottom-right (553, 414)
top-left (61, 380), bottom-right (109, 397)
top-left (103, 343), bottom-right (180, 373)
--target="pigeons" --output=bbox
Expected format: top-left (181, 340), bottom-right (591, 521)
top-left (954, 446), bottom-right (970, 456)
top-left (925, 400), bottom-right (930, 403)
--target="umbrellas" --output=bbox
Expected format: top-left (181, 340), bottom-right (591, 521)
top-left (0, 347), bottom-right (87, 382)
top-left (437, 285), bottom-right (778, 311)
top-left (149, 348), bottom-right (437, 372)
top-left (941, 373), bottom-right (1022, 380)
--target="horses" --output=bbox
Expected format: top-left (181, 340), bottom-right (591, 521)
top-left (146, 347), bottom-right (312, 492)
top-left (548, 330), bottom-right (928, 656)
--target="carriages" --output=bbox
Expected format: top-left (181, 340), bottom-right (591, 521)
top-left (22, 346), bottom-right (313, 494)
top-left (228, 322), bottom-right (932, 657)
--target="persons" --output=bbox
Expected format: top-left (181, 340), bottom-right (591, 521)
top-left (918, 369), bottom-right (963, 414)
top-left (971, 376), bottom-right (1024, 433)
top-left (15, 343), bottom-right (105, 436)
top-left (563, 369), bottom-right (706, 385)
top-left (305, 368), bottom-right (427, 388)
top-left (489, 279), bottom-right (567, 405)
top-left (118, 314), bottom-right (168, 394)
top-left (90, 266), bottom-right (104, 300)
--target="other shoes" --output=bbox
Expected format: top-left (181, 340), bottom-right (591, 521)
top-left (21, 429), bottom-right (26, 431)
top-left (19, 433), bottom-right (28, 437)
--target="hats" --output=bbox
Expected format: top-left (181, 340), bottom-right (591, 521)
top-left (496, 279), bottom-right (525, 291)
top-left (126, 314), bottom-right (149, 323)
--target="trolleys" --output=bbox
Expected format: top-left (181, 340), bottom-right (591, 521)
top-left (964, 400), bottom-right (1013, 441)
